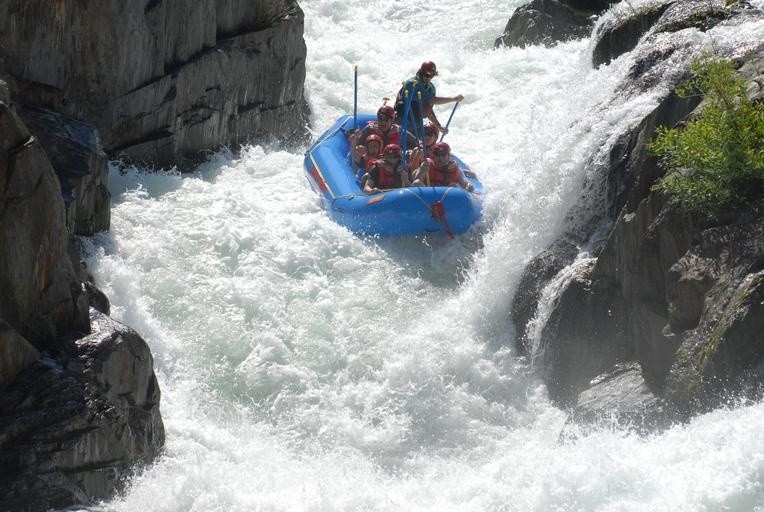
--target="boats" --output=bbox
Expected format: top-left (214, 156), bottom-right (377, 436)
top-left (302, 112), bottom-right (484, 240)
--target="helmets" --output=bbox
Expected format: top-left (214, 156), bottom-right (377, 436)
top-left (365, 134), bottom-right (402, 157)
top-left (423, 122), bottom-right (451, 154)
top-left (421, 60), bottom-right (436, 75)
top-left (377, 105), bottom-right (395, 118)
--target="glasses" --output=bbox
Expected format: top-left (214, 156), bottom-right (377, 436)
top-left (424, 74), bottom-right (434, 78)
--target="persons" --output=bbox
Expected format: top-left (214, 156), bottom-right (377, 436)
top-left (394, 60), bottom-right (465, 135)
top-left (347, 106), bottom-right (474, 194)
